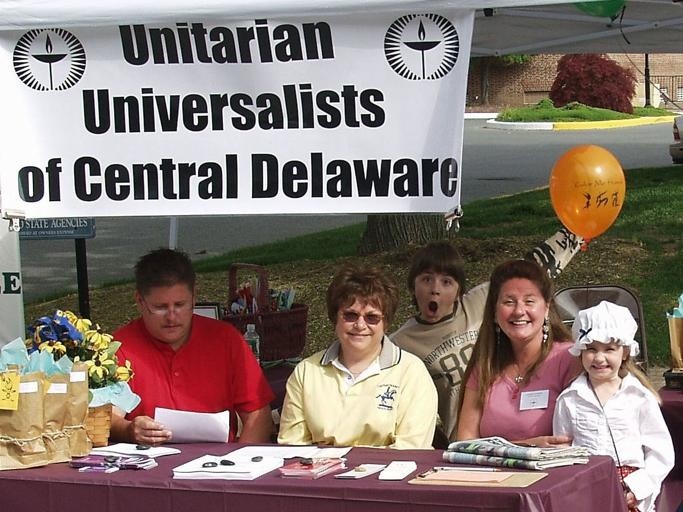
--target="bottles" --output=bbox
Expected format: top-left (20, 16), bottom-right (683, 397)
top-left (242, 324), bottom-right (259, 368)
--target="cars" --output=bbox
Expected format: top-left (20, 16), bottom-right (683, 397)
top-left (668, 112), bottom-right (683, 166)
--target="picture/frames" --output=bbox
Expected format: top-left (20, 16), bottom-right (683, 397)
top-left (193, 301), bottom-right (223, 320)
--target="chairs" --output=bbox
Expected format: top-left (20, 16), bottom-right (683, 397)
top-left (552, 284), bottom-right (648, 372)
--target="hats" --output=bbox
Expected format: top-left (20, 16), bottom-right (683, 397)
top-left (568, 300), bottom-right (640, 357)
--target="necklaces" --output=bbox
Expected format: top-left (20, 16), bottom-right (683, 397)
top-left (509, 342), bottom-right (543, 383)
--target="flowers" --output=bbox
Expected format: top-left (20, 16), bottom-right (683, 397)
top-left (25, 307), bottom-right (135, 388)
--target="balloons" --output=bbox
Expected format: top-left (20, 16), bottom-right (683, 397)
top-left (549, 141), bottom-right (627, 253)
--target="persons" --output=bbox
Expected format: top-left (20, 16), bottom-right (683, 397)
top-left (384, 227), bottom-right (588, 444)
top-left (277, 259), bottom-right (439, 450)
top-left (109, 247), bottom-right (278, 444)
top-left (552, 298), bottom-right (676, 512)
top-left (457, 257), bottom-right (586, 449)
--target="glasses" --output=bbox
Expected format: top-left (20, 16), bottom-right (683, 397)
top-left (141, 295), bottom-right (194, 316)
top-left (337, 310), bottom-right (386, 324)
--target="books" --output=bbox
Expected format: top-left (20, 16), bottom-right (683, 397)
top-left (277, 456), bottom-right (344, 476)
top-left (279, 464), bottom-right (344, 480)
